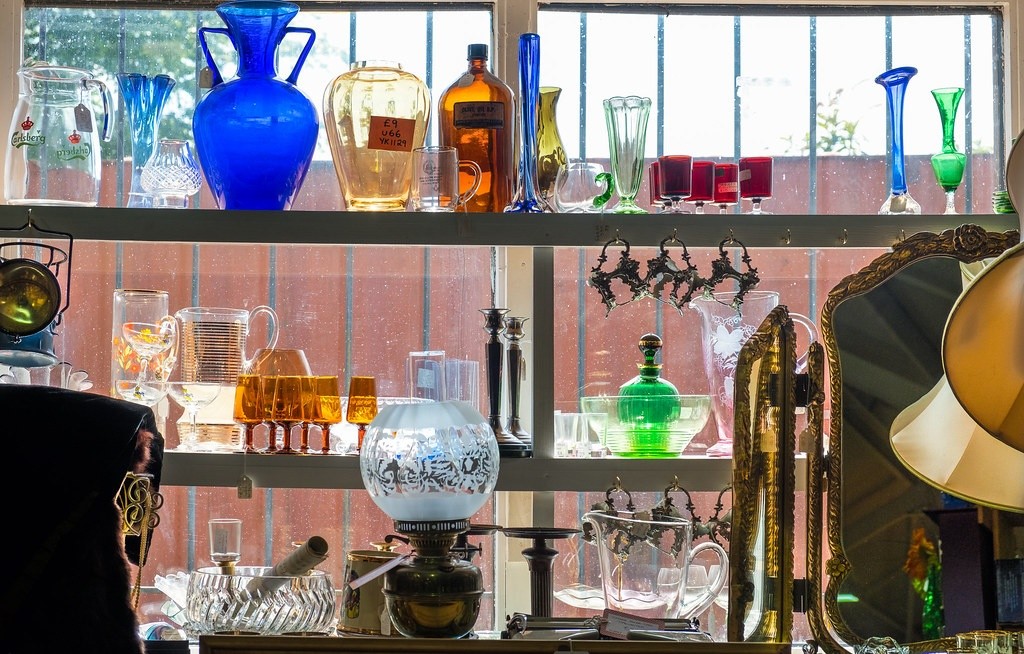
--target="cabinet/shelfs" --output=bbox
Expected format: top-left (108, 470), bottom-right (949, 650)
top-left (0, 0), bottom-right (1024, 654)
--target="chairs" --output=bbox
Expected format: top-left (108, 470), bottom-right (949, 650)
top-left (729, 224), bottom-right (1024, 654)
top-left (0, 383), bottom-right (164, 654)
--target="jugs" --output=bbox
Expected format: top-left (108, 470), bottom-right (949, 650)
top-left (3, 66), bottom-right (115, 207)
top-left (175, 305), bottom-right (280, 422)
top-left (581, 509), bottom-right (729, 623)
top-left (688, 290), bottom-right (818, 457)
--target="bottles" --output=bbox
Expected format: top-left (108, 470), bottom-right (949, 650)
top-left (111, 289), bottom-right (169, 450)
top-left (616, 334), bottom-right (681, 454)
top-left (930, 86), bottom-right (966, 213)
top-left (140, 140), bottom-right (203, 197)
top-left (191, 0), bottom-right (567, 215)
top-left (874, 67), bottom-right (923, 215)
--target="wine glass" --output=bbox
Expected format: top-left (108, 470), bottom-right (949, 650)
top-left (167, 381), bottom-right (221, 453)
top-left (117, 322), bottom-right (175, 395)
top-left (346, 375), bottom-right (378, 455)
top-left (648, 154), bottom-right (775, 215)
top-left (657, 564), bottom-right (729, 643)
top-left (233, 347), bottom-right (342, 456)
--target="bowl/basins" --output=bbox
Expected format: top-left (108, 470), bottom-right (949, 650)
top-left (329, 397), bottom-right (435, 447)
top-left (581, 394), bottom-right (711, 458)
top-left (186, 565), bottom-right (334, 637)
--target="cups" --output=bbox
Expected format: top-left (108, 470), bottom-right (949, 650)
top-left (207, 519), bottom-right (242, 565)
top-left (334, 549), bottom-right (400, 636)
top-left (956, 631), bottom-right (1024, 654)
top-left (116, 379), bottom-right (170, 407)
top-left (114, 74), bottom-right (176, 209)
top-left (412, 144), bottom-right (482, 213)
top-left (552, 97), bottom-right (652, 214)
top-left (554, 410), bottom-right (608, 459)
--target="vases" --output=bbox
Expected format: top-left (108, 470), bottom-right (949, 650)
top-left (503, 33), bottom-right (556, 214)
top-left (191, 0), bottom-right (319, 211)
top-left (116, 73), bottom-right (176, 208)
top-left (539, 86), bottom-right (570, 203)
top-left (874, 66), bottom-right (922, 215)
top-left (930, 86), bottom-right (968, 216)
top-left (603, 95), bottom-right (652, 215)
